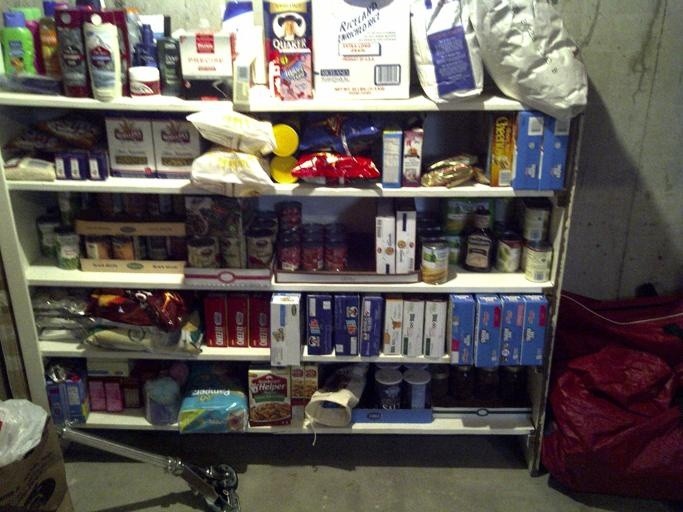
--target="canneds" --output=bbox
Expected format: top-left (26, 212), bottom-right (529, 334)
top-left (37, 192), bottom-right (175, 272)
top-left (416, 208), bottom-right (449, 287)
top-left (188, 198), bottom-right (352, 275)
top-left (496, 196), bottom-right (551, 284)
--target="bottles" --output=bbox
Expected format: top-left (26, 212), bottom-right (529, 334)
top-left (433, 363), bottom-right (526, 408)
top-left (462, 210), bottom-right (497, 274)
top-left (128, 65), bottom-right (161, 98)
top-left (136, 24), bottom-right (160, 67)
top-left (157, 14), bottom-right (185, 97)
top-left (375, 362), bottom-right (432, 410)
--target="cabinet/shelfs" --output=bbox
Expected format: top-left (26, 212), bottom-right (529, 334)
top-left (1, 91), bottom-right (585, 478)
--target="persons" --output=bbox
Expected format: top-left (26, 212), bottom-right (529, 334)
top-left (277, 14), bottom-right (303, 42)
